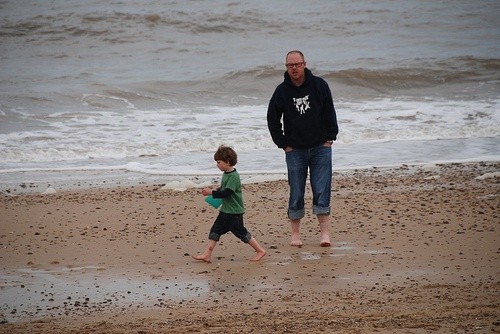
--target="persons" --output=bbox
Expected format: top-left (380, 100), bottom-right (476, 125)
top-left (192, 146), bottom-right (267, 264)
top-left (266, 51), bottom-right (339, 247)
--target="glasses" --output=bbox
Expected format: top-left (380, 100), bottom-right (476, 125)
top-left (286, 62), bottom-right (304, 67)
